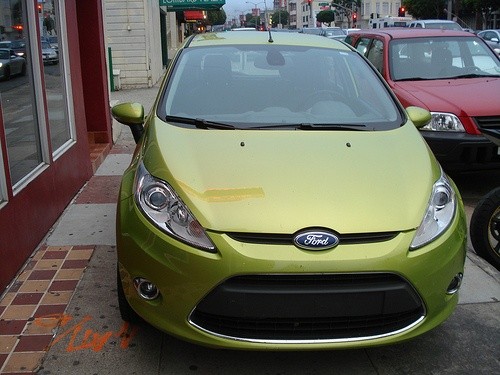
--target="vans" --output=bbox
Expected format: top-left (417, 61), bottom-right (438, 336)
top-left (368, 16), bottom-right (413, 29)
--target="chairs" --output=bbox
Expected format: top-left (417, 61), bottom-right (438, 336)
top-left (427, 50), bottom-right (452, 78)
top-left (203, 55), bottom-right (233, 91)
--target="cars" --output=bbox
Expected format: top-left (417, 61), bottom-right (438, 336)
top-left (110, 30), bottom-right (469, 353)
top-left (474, 29), bottom-right (500, 57)
top-left (0, 36), bottom-right (58, 81)
top-left (301, 26), bottom-right (362, 42)
top-left (408, 19), bottom-right (500, 75)
top-left (343, 25), bottom-right (500, 170)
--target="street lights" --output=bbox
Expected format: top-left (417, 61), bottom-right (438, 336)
top-left (245, 1), bottom-right (265, 27)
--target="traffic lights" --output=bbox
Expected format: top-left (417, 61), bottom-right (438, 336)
top-left (352, 13), bottom-right (357, 23)
top-left (370, 13), bottom-right (373, 19)
top-left (400, 7), bottom-right (405, 17)
top-left (38, 4), bottom-right (42, 13)
top-left (377, 14), bottom-right (380, 18)
